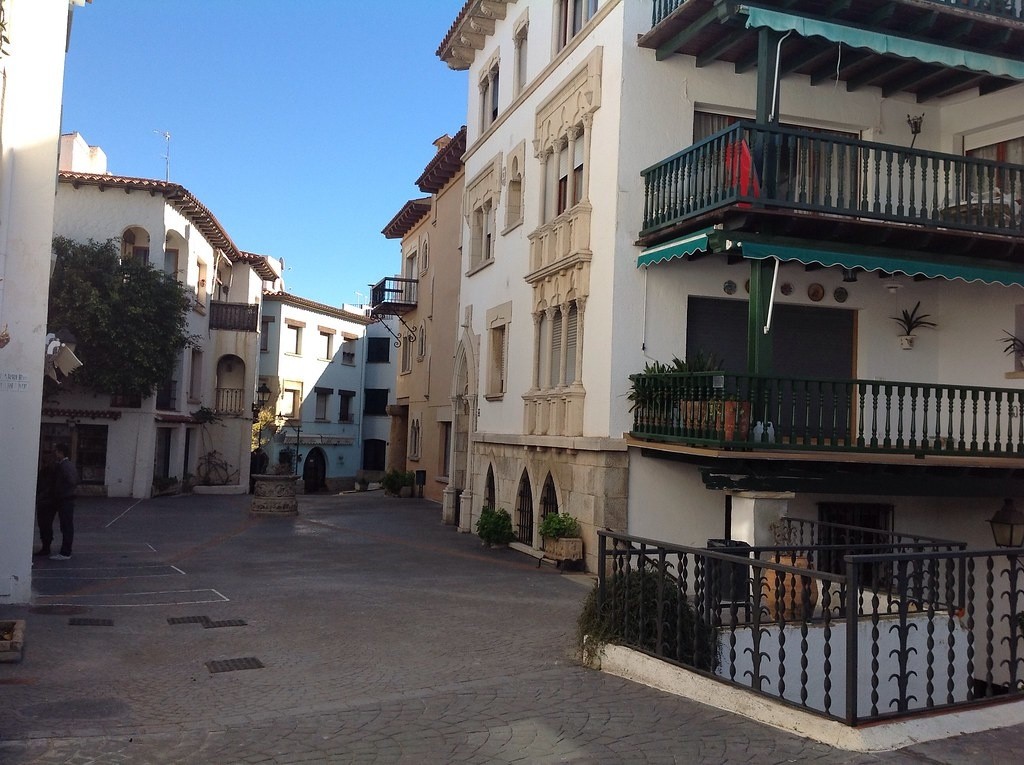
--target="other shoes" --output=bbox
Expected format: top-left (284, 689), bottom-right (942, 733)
top-left (33, 551), bottom-right (51, 557)
top-left (50, 553), bottom-right (72, 560)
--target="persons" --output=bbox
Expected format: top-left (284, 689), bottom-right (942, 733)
top-left (34, 451), bottom-right (59, 555)
top-left (47, 443), bottom-right (83, 560)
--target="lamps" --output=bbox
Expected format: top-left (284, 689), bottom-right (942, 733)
top-left (904, 112), bottom-right (926, 158)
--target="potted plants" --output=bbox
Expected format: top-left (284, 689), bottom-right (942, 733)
top-left (383, 467), bottom-right (415, 498)
top-left (996, 329), bottom-right (1024, 369)
top-left (475, 506), bottom-right (518, 550)
top-left (624, 353), bottom-right (750, 445)
top-left (538, 512), bottom-right (583, 560)
top-left (888, 301), bottom-right (937, 350)
